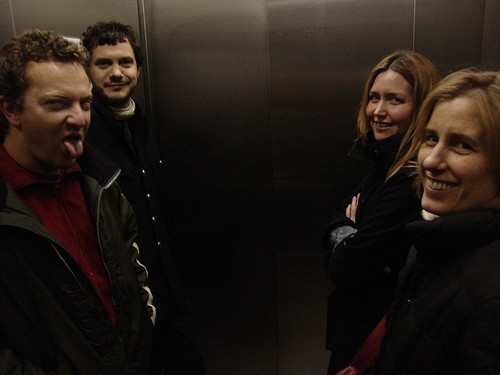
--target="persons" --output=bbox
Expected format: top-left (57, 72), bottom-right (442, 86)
top-left (323, 50), bottom-right (442, 374)
top-left (0, 28), bottom-right (158, 375)
top-left (337, 64), bottom-right (500, 375)
top-left (82, 23), bottom-right (206, 375)
